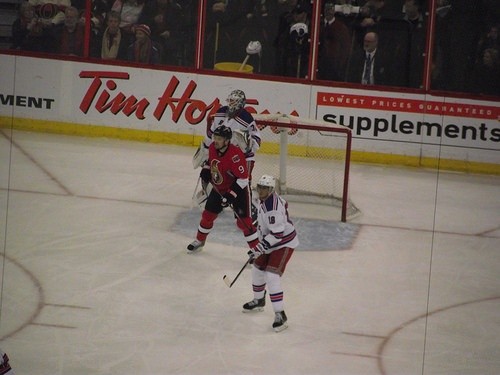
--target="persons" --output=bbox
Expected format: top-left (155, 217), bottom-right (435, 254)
top-left (242, 173), bottom-right (299, 334)
top-left (196, 89), bottom-right (261, 204)
top-left (11, 0), bottom-right (500, 68)
top-left (419, 49), bottom-right (459, 92)
top-left (343, 29), bottom-right (406, 86)
top-left (187, 124), bottom-right (260, 269)
top-left (468, 48), bottom-right (500, 95)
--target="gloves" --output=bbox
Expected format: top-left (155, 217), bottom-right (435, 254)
top-left (247, 241), bottom-right (268, 264)
top-left (200, 168), bottom-right (211, 183)
top-left (217, 192), bottom-right (237, 208)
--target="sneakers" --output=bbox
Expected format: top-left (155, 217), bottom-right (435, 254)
top-left (186, 239), bottom-right (206, 254)
top-left (272, 310), bottom-right (288, 332)
top-left (242, 299), bottom-right (266, 313)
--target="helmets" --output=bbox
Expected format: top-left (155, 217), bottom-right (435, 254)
top-left (214, 125), bottom-right (232, 139)
top-left (291, 22), bottom-right (312, 43)
top-left (257, 175), bottom-right (275, 188)
top-left (228, 90), bottom-right (246, 112)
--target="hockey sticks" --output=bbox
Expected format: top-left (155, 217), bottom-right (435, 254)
top-left (222, 255), bottom-right (254, 288)
top-left (191, 174), bottom-right (201, 210)
top-left (210, 181), bottom-right (253, 233)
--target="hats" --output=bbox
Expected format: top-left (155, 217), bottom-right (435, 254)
top-left (291, 4), bottom-right (305, 14)
top-left (136, 24), bottom-right (151, 37)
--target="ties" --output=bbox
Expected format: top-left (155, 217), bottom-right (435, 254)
top-left (363, 54), bottom-right (370, 85)
